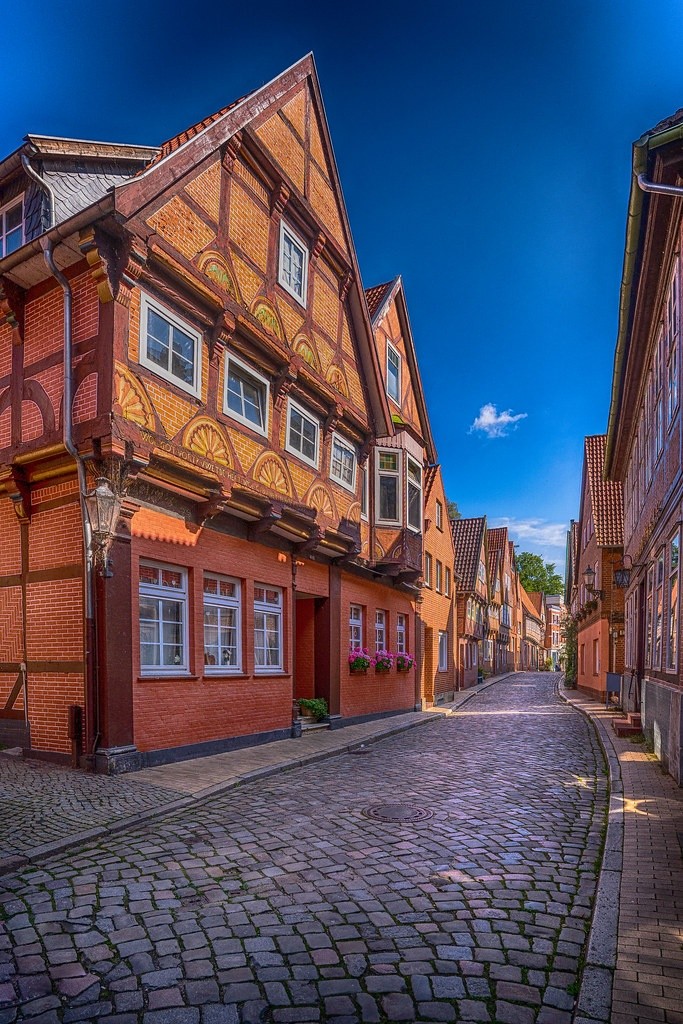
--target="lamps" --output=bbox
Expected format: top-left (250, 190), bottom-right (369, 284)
top-left (81, 476), bottom-right (121, 579)
top-left (613, 555), bottom-right (646, 589)
top-left (582, 564), bottom-right (604, 600)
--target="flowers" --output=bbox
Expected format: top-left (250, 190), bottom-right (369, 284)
top-left (348, 647), bottom-right (416, 671)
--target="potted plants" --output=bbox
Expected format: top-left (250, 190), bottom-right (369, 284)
top-left (298, 697), bottom-right (328, 720)
